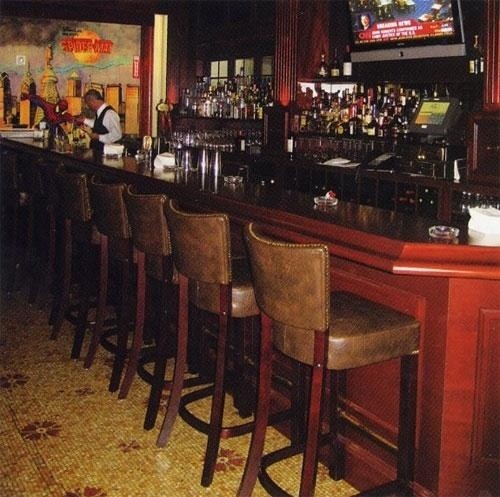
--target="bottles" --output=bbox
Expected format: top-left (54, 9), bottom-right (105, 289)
top-left (287, 121), bottom-right (296, 158)
top-left (181, 66), bottom-right (272, 119)
top-left (318, 45), bottom-right (352, 76)
top-left (466, 34), bottom-right (485, 76)
top-left (298, 83), bottom-right (455, 141)
top-left (235, 125), bottom-right (246, 153)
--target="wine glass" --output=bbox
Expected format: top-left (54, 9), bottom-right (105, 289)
top-left (459, 191), bottom-right (500, 214)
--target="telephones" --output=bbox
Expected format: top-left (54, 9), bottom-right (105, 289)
top-left (360, 148), bottom-right (398, 170)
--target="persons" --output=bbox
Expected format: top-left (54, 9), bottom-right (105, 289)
top-left (357, 13), bottom-right (372, 32)
top-left (20, 90), bottom-right (84, 139)
top-left (80, 88), bottom-right (124, 151)
top-left (81, 149), bottom-right (125, 169)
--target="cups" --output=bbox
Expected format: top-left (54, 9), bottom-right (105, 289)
top-left (298, 136), bottom-right (378, 156)
top-left (175, 124), bottom-right (263, 147)
top-left (180, 148), bottom-right (223, 175)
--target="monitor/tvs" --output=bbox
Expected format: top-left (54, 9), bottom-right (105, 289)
top-left (347, 0), bottom-right (467, 63)
top-left (408, 97), bottom-right (461, 136)
